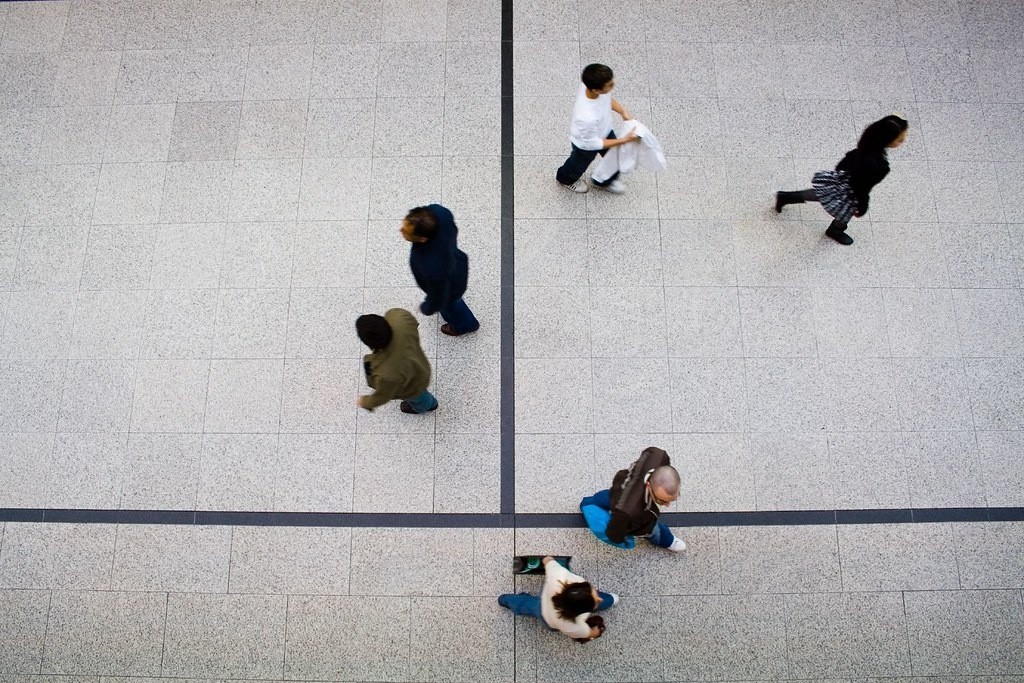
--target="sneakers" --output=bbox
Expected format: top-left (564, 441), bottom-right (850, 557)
top-left (590, 178), bottom-right (626, 195)
top-left (556, 178), bottom-right (589, 193)
top-left (668, 536), bottom-right (687, 552)
top-left (607, 593), bottom-right (619, 606)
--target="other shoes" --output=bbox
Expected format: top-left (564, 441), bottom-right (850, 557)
top-left (497, 592), bottom-right (530, 610)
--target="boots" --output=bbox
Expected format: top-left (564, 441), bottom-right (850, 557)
top-left (825, 219), bottom-right (854, 246)
top-left (774, 191), bottom-right (805, 214)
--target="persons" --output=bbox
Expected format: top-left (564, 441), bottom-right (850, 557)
top-left (400, 204), bottom-right (480, 337)
top-left (496, 557), bottom-right (619, 640)
top-left (356, 308), bottom-right (439, 414)
top-left (608, 446), bottom-right (688, 553)
top-left (774, 116), bottom-right (910, 245)
top-left (556, 63), bottom-right (641, 195)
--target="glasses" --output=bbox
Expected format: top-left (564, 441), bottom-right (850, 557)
top-left (649, 482), bottom-right (668, 506)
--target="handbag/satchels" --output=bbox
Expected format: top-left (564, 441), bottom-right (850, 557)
top-left (512, 555), bottom-right (573, 575)
top-left (572, 615), bottom-right (605, 645)
top-left (579, 490), bottom-right (636, 550)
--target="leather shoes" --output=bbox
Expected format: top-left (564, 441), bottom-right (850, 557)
top-left (420, 302), bottom-right (435, 316)
top-left (441, 321), bottom-right (479, 336)
top-left (400, 401), bottom-right (438, 414)
